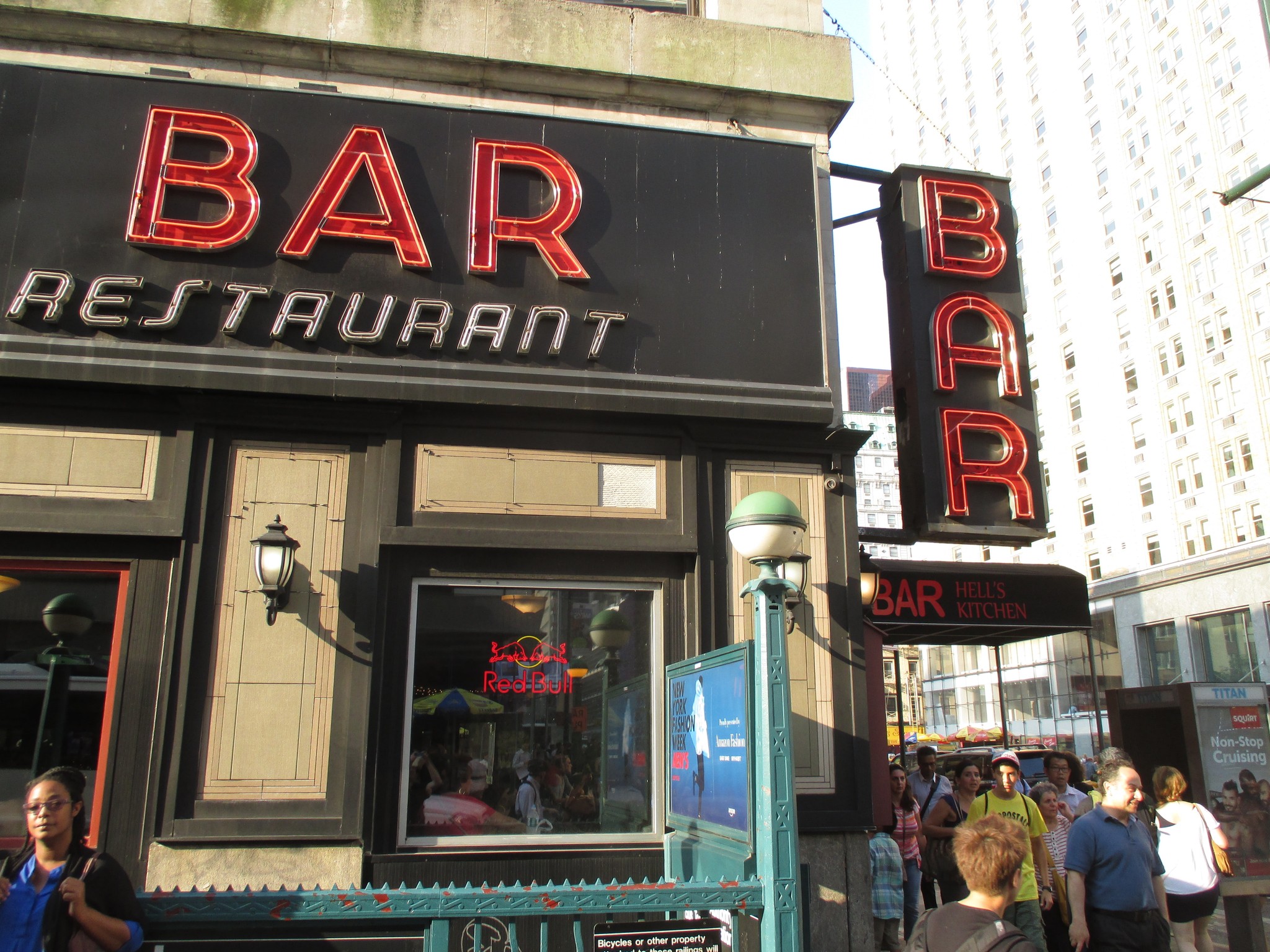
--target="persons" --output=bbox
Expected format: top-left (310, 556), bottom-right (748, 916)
top-left (0, 767), bottom-right (147, 952)
top-left (1068, 704), bottom-right (1078, 714)
top-left (1208, 769), bottom-right (1270, 858)
top-left (867, 745), bottom-right (1230, 952)
top-left (408, 733), bottom-right (649, 834)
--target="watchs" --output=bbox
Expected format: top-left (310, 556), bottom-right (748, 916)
top-left (1042, 885), bottom-right (1053, 893)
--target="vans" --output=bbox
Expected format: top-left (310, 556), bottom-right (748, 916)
top-left (0, 663), bottom-right (111, 837)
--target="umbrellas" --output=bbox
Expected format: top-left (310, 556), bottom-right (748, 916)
top-left (414, 689), bottom-right (503, 717)
top-left (904, 726), bottom-right (1012, 745)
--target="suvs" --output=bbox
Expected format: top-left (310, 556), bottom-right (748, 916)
top-left (889, 743), bottom-right (1056, 796)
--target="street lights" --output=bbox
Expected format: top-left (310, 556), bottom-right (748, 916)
top-left (589, 607), bottom-right (632, 834)
top-left (725, 489), bottom-right (809, 952)
top-left (30, 594), bottom-right (100, 779)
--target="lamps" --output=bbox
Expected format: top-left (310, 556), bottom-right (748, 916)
top-left (777, 548), bottom-right (812, 633)
top-left (249, 514), bottom-right (301, 628)
top-left (859, 543), bottom-right (882, 614)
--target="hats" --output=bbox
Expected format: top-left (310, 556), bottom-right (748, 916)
top-left (991, 750), bottom-right (1019, 767)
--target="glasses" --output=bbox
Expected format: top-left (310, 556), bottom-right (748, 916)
top-left (23, 799), bottom-right (73, 814)
top-left (920, 762), bottom-right (936, 767)
top-left (1049, 766), bottom-right (1069, 773)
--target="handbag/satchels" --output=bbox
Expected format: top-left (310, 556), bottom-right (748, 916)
top-left (925, 793), bottom-right (966, 886)
top-left (1193, 802), bottom-right (1234, 877)
top-left (68, 852), bottom-right (108, 952)
top-left (901, 862), bottom-right (907, 881)
top-left (1051, 870), bottom-right (1070, 927)
